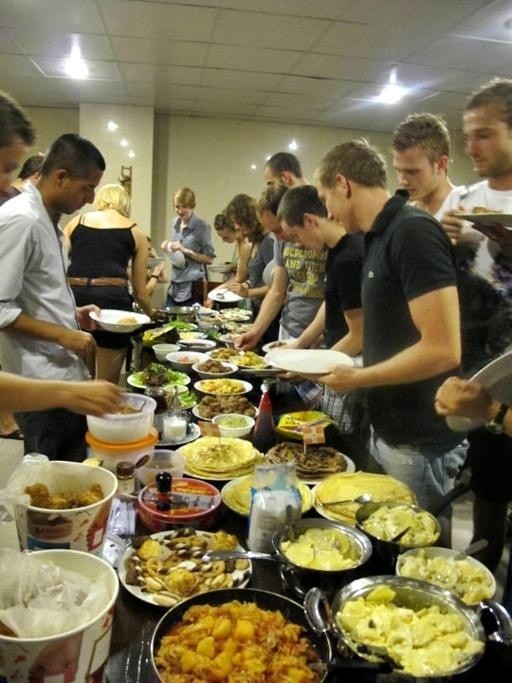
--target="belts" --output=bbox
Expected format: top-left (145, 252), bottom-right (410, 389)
top-left (67, 276), bottom-right (127, 287)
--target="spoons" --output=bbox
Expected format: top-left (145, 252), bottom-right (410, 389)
top-left (216, 289), bottom-right (231, 300)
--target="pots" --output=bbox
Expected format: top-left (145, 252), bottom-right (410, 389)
top-left (200, 518), bottom-right (375, 584)
top-left (355, 479), bottom-right (471, 551)
top-left (298, 571), bottom-right (512, 683)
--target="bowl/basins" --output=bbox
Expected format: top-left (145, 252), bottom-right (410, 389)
top-left (211, 412), bottom-right (257, 437)
top-left (395, 538), bottom-right (498, 605)
top-left (148, 585), bottom-right (333, 683)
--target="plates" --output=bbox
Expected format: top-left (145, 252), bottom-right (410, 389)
top-left (191, 376), bottom-right (254, 396)
top-left (207, 288), bottom-right (245, 304)
top-left (191, 395), bottom-right (261, 422)
top-left (444, 351), bottom-right (512, 434)
top-left (219, 471), bottom-right (314, 523)
top-left (125, 371), bottom-right (191, 390)
top-left (311, 470), bottom-right (419, 528)
top-left (265, 443), bottom-right (356, 485)
top-left (151, 305), bottom-right (288, 379)
top-left (113, 527), bottom-right (256, 607)
top-left (204, 261), bottom-right (234, 274)
top-left (262, 337), bottom-right (358, 377)
top-left (453, 213), bottom-right (512, 229)
top-left (169, 436), bottom-right (266, 482)
top-left (149, 414), bottom-right (202, 449)
top-left (88, 305), bottom-right (151, 334)
top-left (163, 240), bottom-right (186, 269)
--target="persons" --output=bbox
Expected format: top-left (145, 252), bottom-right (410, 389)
top-left (1, 92), bottom-right (164, 462)
top-left (159, 187), bottom-right (216, 310)
top-left (205, 78), bottom-right (512, 612)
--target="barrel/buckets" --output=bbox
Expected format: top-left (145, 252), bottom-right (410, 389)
top-left (0, 547), bottom-right (121, 683)
top-left (12, 460), bottom-right (117, 554)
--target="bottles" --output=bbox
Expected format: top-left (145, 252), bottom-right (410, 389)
top-left (161, 386), bottom-right (188, 444)
top-left (114, 454), bottom-right (150, 496)
